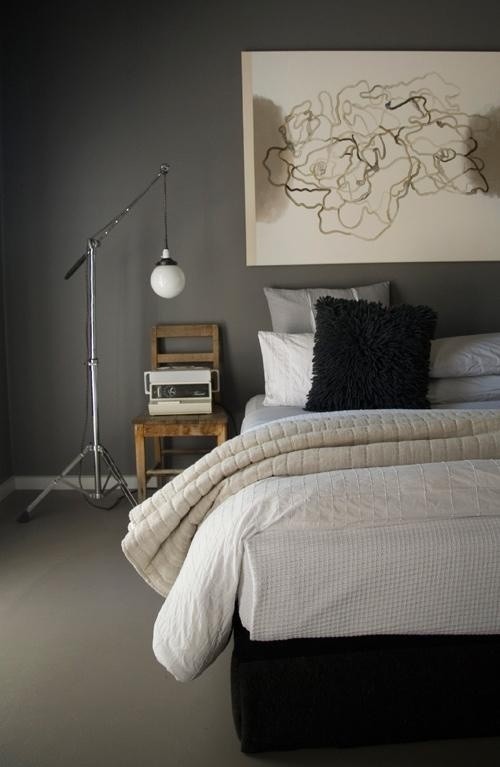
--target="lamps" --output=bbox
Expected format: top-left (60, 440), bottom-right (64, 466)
top-left (14, 163), bottom-right (186, 524)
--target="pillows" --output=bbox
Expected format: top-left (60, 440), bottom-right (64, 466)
top-left (258, 330), bottom-right (319, 408)
top-left (422, 332), bottom-right (500, 379)
top-left (425, 375), bottom-right (500, 404)
top-left (262, 281), bottom-right (391, 334)
top-left (303, 295), bottom-right (437, 411)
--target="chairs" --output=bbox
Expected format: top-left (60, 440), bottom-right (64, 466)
top-left (132, 323), bottom-right (229, 504)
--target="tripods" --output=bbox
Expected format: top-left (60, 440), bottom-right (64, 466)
top-left (19, 249), bottom-right (139, 522)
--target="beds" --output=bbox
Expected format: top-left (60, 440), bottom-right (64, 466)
top-left (234, 394), bottom-right (500, 753)
